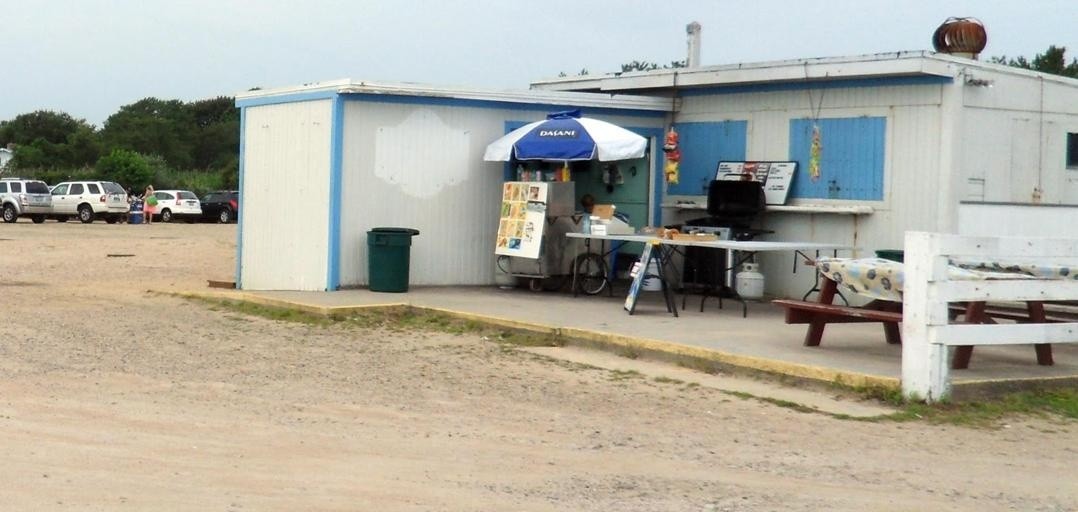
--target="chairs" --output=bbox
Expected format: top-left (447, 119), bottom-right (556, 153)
top-left (661, 181), bottom-right (766, 314)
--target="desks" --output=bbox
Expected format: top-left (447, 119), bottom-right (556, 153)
top-left (566, 234), bottom-right (861, 316)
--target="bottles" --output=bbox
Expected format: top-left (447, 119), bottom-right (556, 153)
top-left (517, 165), bottom-right (546, 181)
top-left (556, 165), bottom-right (571, 182)
top-left (583, 210), bottom-right (590, 234)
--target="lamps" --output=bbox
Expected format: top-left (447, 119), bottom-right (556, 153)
top-left (968, 78), bottom-right (993, 88)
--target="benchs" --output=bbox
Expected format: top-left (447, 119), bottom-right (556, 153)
top-left (771, 251), bottom-right (1078, 370)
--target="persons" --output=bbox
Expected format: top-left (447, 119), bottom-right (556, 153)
top-left (141, 184), bottom-right (159, 224)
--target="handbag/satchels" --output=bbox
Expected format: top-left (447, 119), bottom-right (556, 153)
top-left (146, 196), bottom-right (158, 206)
top-left (707, 173), bottom-right (765, 217)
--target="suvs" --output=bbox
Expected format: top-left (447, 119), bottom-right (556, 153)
top-left (0, 177), bottom-right (240, 225)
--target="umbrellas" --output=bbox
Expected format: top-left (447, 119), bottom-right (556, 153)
top-left (483, 108), bottom-right (648, 169)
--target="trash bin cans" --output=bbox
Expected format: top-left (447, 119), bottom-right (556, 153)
top-left (367, 226), bottom-right (420, 292)
top-left (126, 200), bottom-right (143, 224)
